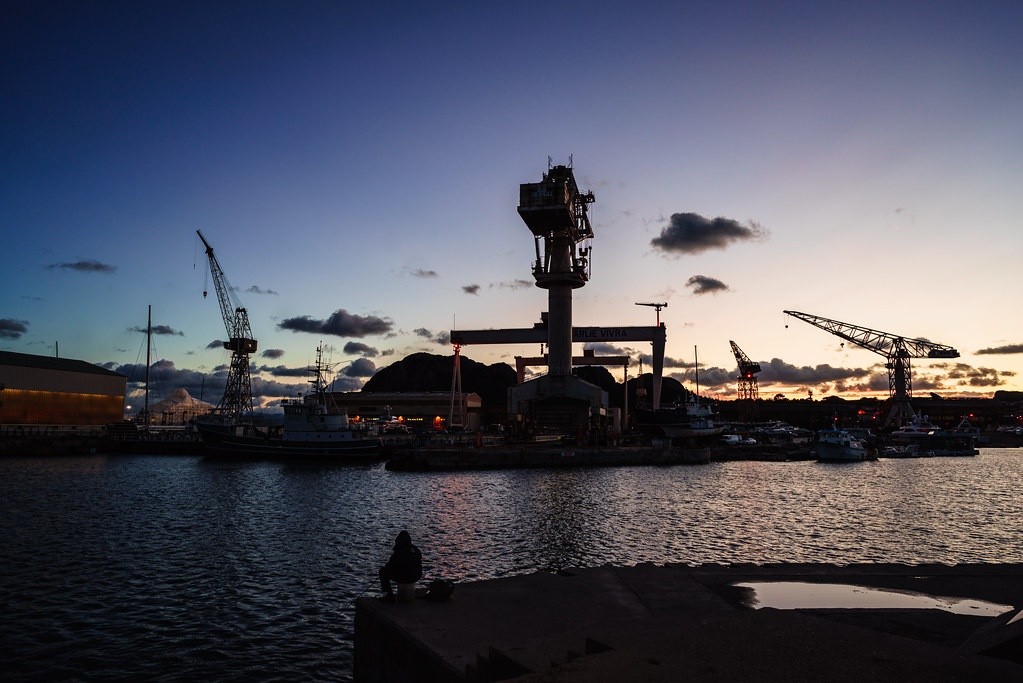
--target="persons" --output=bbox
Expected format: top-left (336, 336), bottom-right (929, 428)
top-left (378, 530), bottom-right (423, 598)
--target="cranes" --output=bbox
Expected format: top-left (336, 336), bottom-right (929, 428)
top-left (193, 228), bottom-right (259, 430)
top-left (729, 338), bottom-right (763, 399)
top-left (784, 308), bottom-right (960, 429)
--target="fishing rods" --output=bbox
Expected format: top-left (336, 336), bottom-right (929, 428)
top-left (303, 575), bottom-right (380, 648)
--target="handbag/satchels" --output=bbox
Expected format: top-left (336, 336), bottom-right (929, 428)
top-left (430, 578), bottom-right (443, 601)
top-left (440, 579), bottom-right (457, 601)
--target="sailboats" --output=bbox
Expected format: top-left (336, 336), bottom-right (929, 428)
top-left (84, 305), bottom-right (171, 448)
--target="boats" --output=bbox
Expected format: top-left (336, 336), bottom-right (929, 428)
top-left (633, 389), bottom-right (980, 457)
top-left (166, 421), bottom-right (266, 463)
top-left (384, 435), bottom-right (564, 473)
top-left (198, 348), bottom-right (384, 455)
top-left (570, 383), bottom-right (729, 466)
